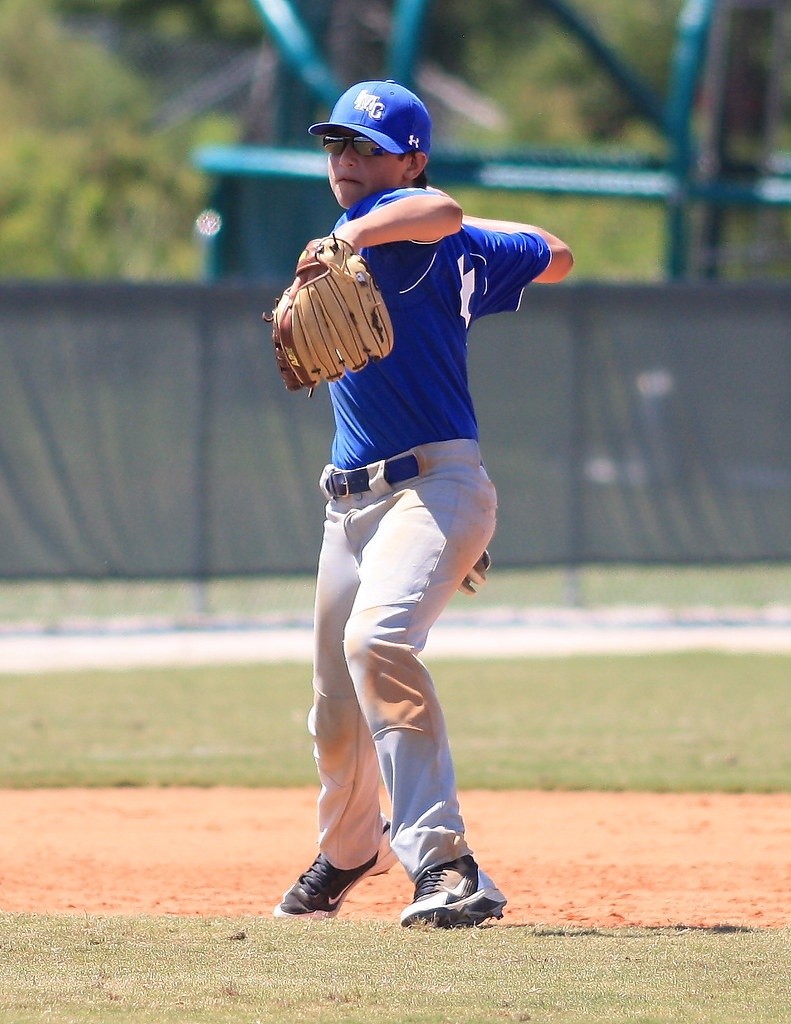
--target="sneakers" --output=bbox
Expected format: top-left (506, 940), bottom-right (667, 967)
top-left (274, 814), bottom-right (398, 919)
top-left (400, 853), bottom-right (506, 928)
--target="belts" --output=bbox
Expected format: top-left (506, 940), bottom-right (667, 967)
top-left (326, 453), bottom-right (419, 497)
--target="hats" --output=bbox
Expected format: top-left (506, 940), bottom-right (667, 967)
top-left (307, 79), bottom-right (431, 155)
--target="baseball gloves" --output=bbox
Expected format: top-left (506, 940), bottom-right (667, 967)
top-left (273, 236), bottom-right (390, 391)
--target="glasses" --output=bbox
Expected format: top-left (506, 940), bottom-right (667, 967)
top-left (323, 134), bottom-right (383, 157)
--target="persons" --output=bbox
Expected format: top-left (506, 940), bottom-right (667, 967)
top-left (272, 79), bottom-right (574, 927)
